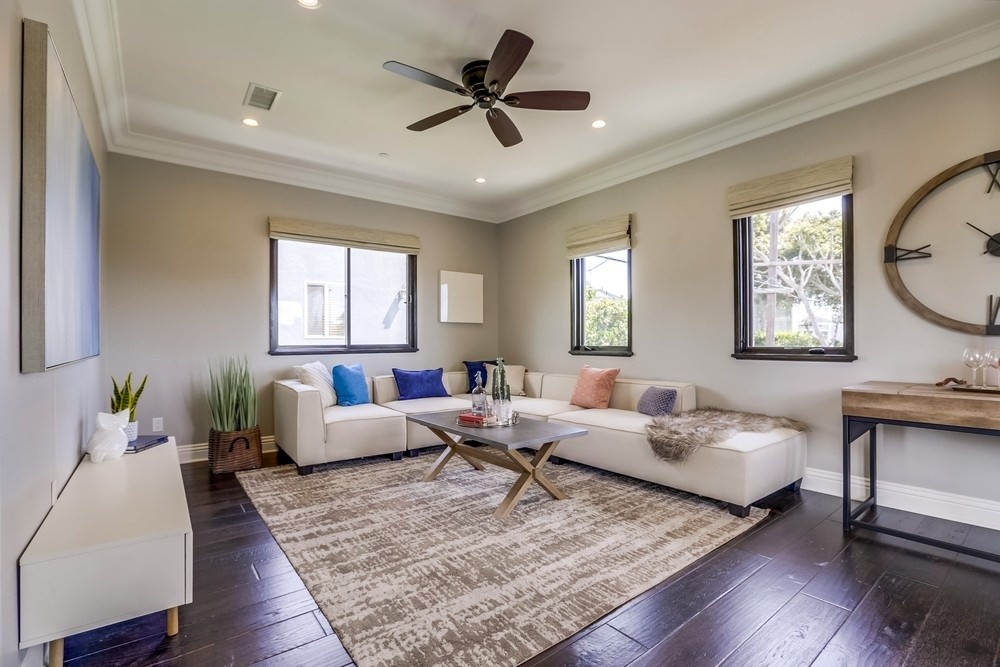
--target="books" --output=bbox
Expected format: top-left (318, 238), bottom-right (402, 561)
top-left (123, 436), bottom-right (168, 454)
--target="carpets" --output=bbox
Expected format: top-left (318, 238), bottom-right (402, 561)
top-left (235, 439), bottom-right (772, 667)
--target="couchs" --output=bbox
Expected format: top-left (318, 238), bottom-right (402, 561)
top-left (272, 371), bottom-right (812, 518)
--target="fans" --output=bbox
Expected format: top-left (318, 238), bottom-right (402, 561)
top-left (383, 30), bottom-right (591, 147)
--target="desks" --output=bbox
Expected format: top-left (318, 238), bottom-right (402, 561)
top-left (841, 381), bottom-right (1000, 563)
top-left (406, 409), bottom-right (588, 517)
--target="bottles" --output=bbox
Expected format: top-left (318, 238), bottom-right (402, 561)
top-left (472, 371), bottom-right (488, 427)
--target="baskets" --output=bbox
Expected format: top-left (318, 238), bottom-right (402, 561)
top-left (207, 424), bottom-right (263, 474)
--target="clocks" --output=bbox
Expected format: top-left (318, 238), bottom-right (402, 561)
top-left (883, 151), bottom-right (1000, 336)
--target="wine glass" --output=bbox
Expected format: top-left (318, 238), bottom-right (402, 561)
top-left (963, 348), bottom-right (986, 388)
top-left (980, 349), bottom-right (991, 389)
top-left (990, 348), bottom-right (1000, 387)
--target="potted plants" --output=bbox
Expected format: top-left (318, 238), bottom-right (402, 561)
top-left (205, 355), bottom-right (262, 473)
top-left (110, 371), bottom-right (148, 441)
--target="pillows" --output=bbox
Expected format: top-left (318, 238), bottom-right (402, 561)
top-left (637, 386), bottom-right (677, 416)
top-left (392, 368), bottom-right (452, 400)
top-left (293, 361), bottom-right (337, 408)
top-left (569, 365), bottom-right (620, 409)
top-left (481, 363), bottom-right (528, 395)
top-left (462, 360), bottom-right (505, 394)
top-left (333, 363), bottom-right (370, 406)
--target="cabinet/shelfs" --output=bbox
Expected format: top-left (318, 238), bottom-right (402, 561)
top-left (20, 436), bottom-right (194, 667)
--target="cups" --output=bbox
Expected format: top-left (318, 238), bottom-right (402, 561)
top-left (487, 400), bottom-right (513, 425)
